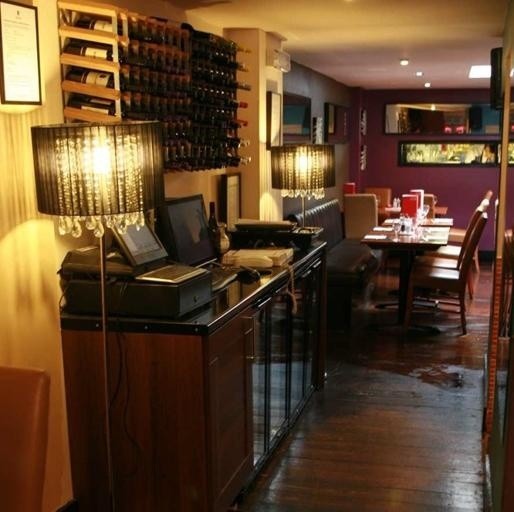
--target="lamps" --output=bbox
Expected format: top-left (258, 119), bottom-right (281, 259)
top-left (271, 143), bottom-right (336, 228)
top-left (31, 120), bottom-right (168, 512)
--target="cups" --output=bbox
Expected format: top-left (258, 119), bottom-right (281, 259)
top-left (401, 188), bottom-right (424, 217)
top-left (343, 182), bottom-right (355, 194)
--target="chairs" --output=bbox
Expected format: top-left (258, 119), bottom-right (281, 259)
top-left (405, 191), bottom-right (493, 335)
top-left (0, 365), bottom-right (50, 511)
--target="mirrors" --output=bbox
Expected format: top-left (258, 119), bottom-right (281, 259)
top-left (284, 91), bottom-right (312, 145)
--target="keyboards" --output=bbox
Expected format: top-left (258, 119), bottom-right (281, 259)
top-left (209, 268), bottom-right (238, 292)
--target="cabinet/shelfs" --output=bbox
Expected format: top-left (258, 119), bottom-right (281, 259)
top-left (59, 238), bottom-right (328, 510)
top-left (59, 0), bottom-right (238, 173)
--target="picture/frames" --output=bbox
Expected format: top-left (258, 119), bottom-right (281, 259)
top-left (324, 102), bottom-right (336, 135)
top-left (397, 141), bottom-right (514, 168)
top-left (266, 91), bottom-right (281, 150)
top-left (0, 0), bottom-right (42, 106)
top-left (221, 171), bottom-right (241, 233)
top-left (382, 101), bottom-right (514, 137)
top-left (110, 218), bottom-right (169, 267)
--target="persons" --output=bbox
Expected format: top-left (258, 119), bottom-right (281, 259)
top-left (475, 144), bottom-right (497, 164)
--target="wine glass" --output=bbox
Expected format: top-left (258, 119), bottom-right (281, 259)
top-left (418, 203), bottom-right (430, 224)
top-left (391, 219), bottom-right (401, 242)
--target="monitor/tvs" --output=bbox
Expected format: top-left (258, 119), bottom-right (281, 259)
top-left (109, 214), bottom-right (169, 266)
top-left (163, 194), bottom-right (218, 268)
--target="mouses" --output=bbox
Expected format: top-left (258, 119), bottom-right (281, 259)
top-left (239, 271), bottom-right (256, 279)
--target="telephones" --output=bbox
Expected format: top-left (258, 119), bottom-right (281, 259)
top-left (234, 255), bottom-right (274, 272)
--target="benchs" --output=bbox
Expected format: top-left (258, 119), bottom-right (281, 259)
top-left (289, 197), bottom-right (372, 327)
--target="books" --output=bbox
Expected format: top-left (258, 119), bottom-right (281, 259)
top-left (222, 247), bottom-right (293, 266)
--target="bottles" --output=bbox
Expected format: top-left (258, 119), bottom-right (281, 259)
top-left (400, 214), bottom-right (411, 233)
top-left (217, 221), bottom-right (230, 253)
top-left (64, 13), bottom-right (253, 172)
top-left (392, 197), bottom-right (401, 208)
top-left (207, 200), bottom-right (221, 263)
